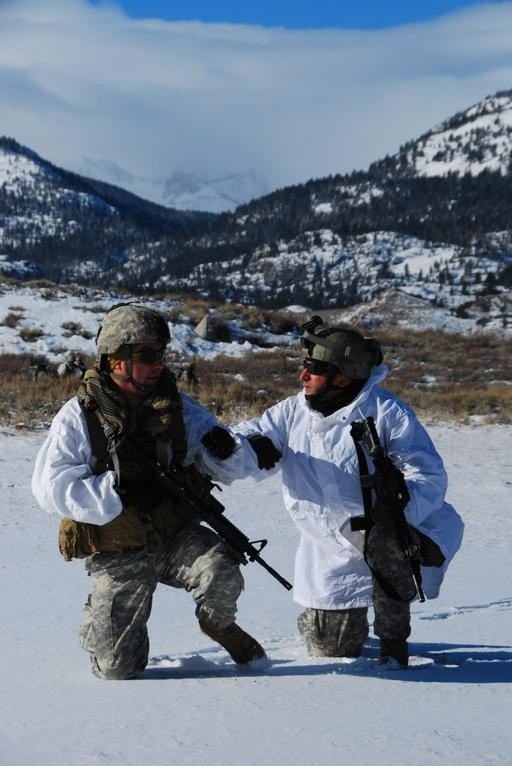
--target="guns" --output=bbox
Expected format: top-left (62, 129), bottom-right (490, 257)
top-left (106, 432), bottom-right (295, 591)
top-left (349, 415), bottom-right (427, 602)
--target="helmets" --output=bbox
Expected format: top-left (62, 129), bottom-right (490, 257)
top-left (302, 316), bottom-right (384, 379)
top-left (97, 305), bottom-right (170, 368)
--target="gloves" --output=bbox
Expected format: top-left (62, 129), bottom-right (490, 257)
top-left (368, 500), bottom-right (397, 524)
top-left (134, 461), bottom-right (169, 498)
top-left (201, 426), bottom-right (235, 460)
top-left (248, 433), bottom-right (282, 471)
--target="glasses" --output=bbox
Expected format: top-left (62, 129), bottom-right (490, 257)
top-left (303, 359), bottom-right (328, 374)
top-left (129, 346), bottom-right (167, 364)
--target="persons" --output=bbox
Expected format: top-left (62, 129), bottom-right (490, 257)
top-left (229, 320), bottom-right (464, 669)
top-left (30, 303), bottom-right (269, 682)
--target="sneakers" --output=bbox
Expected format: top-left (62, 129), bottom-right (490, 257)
top-left (198, 620), bottom-right (268, 670)
top-left (379, 639), bottom-right (408, 670)
